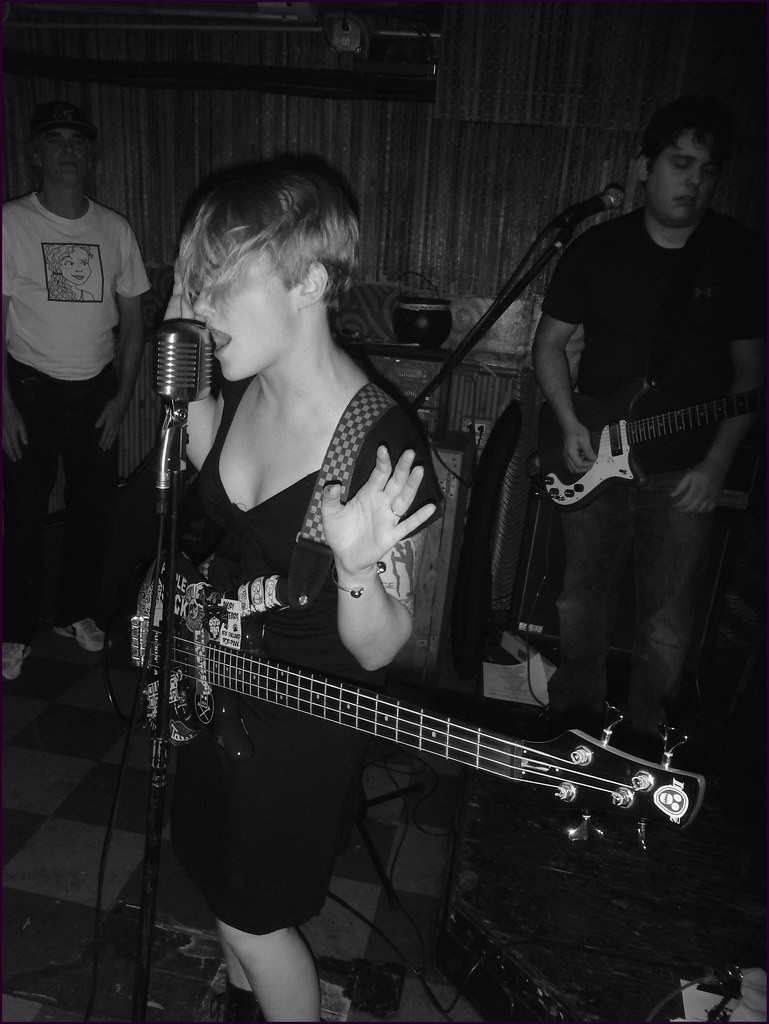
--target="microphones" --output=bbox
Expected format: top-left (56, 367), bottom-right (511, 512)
top-left (552, 186), bottom-right (625, 230)
top-left (150, 318), bottom-right (214, 515)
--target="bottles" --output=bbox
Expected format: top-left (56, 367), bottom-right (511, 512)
top-left (392, 297), bottom-right (453, 351)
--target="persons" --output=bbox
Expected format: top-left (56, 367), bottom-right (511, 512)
top-left (140, 151), bottom-right (447, 1023)
top-left (0, 101), bottom-right (153, 681)
top-left (530, 94), bottom-right (769, 746)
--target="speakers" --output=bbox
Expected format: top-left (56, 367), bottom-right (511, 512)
top-left (508, 474), bottom-right (736, 677)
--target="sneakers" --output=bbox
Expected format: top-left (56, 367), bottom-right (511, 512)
top-left (52, 617), bottom-right (106, 652)
top-left (2, 643), bottom-right (32, 680)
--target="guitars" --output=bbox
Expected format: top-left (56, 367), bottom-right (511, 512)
top-left (535, 375), bottom-right (769, 511)
top-left (132, 538), bottom-right (709, 849)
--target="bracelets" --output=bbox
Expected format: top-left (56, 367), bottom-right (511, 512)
top-left (330, 561), bottom-right (387, 598)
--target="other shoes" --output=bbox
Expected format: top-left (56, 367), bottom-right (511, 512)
top-left (210, 973), bottom-right (266, 1024)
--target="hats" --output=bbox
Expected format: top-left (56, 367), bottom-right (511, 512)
top-left (28, 101), bottom-right (99, 145)
